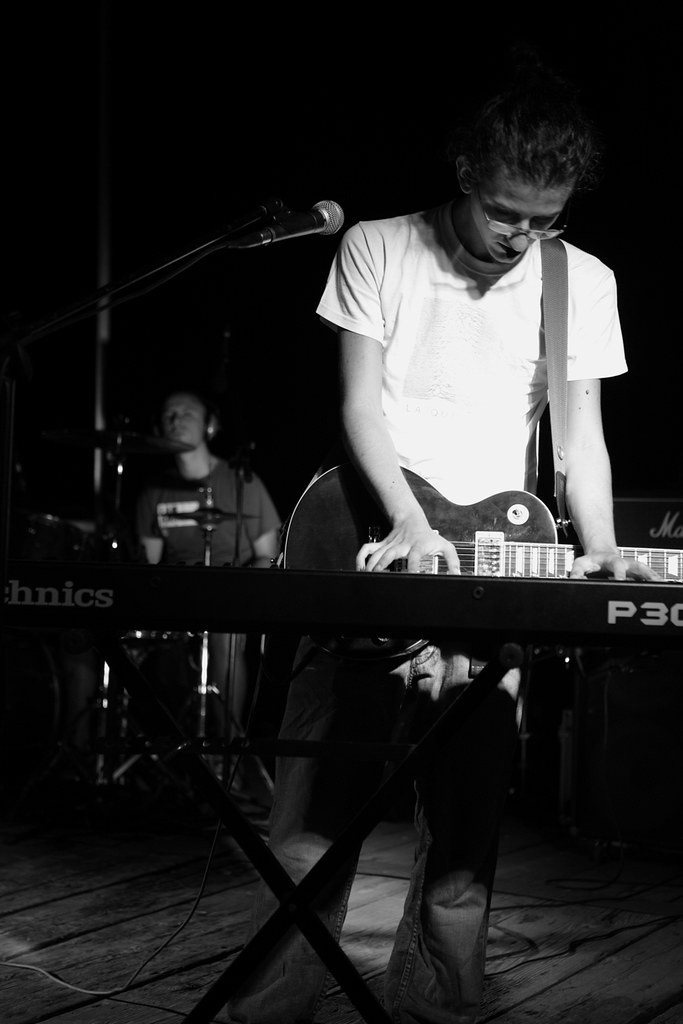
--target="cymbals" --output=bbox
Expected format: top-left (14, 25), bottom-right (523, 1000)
top-left (156, 505), bottom-right (258, 523)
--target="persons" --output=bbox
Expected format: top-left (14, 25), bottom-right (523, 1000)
top-left (133, 391), bottom-right (282, 842)
top-left (227, 97), bottom-right (659, 1023)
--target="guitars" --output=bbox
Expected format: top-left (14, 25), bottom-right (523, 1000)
top-left (280, 452), bottom-right (683, 584)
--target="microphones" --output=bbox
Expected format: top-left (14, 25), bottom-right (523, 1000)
top-left (231, 201), bottom-right (344, 249)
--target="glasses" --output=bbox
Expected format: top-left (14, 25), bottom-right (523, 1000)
top-left (476, 180), bottom-right (571, 240)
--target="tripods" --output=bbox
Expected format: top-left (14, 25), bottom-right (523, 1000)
top-left (175, 528), bottom-right (275, 799)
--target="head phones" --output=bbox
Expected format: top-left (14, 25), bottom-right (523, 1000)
top-left (154, 416), bottom-right (220, 440)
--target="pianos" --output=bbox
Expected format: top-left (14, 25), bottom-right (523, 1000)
top-left (3, 552), bottom-right (683, 656)
